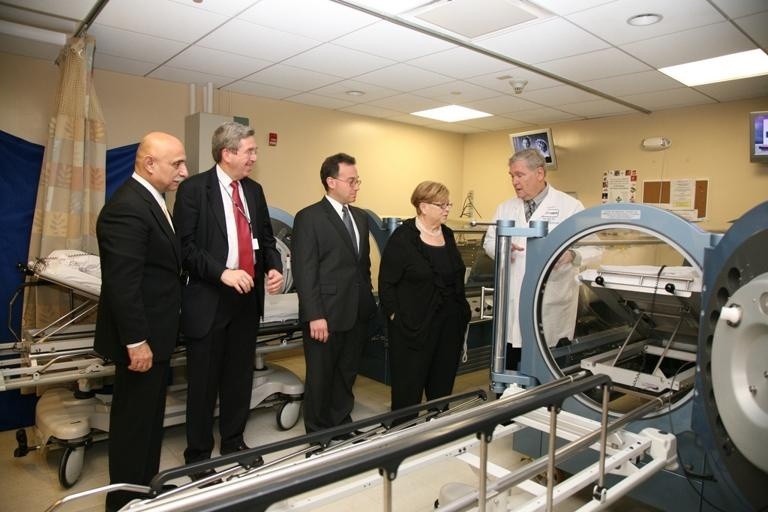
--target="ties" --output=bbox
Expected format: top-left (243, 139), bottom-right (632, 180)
top-left (230, 180), bottom-right (256, 280)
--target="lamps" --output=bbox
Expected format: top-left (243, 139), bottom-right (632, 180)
top-left (626, 10), bottom-right (663, 30)
top-left (345, 90), bottom-right (365, 97)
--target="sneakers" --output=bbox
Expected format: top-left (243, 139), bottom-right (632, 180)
top-left (236, 148), bottom-right (260, 157)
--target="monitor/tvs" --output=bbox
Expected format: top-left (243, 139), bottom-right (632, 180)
top-left (748, 110), bottom-right (768, 163)
top-left (509, 128), bottom-right (557, 172)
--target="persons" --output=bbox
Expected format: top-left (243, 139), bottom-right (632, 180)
top-left (483, 148), bottom-right (604, 371)
top-left (93, 132), bottom-right (187, 512)
top-left (290, 153), bottom-right (374, 458)
top-left (378, 180), bottom-right (472, 428)
top-left (174, 121), bottom-right (283, 488)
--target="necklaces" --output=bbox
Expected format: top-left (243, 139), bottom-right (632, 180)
top-left (414, 217), bottom-right (444, 237)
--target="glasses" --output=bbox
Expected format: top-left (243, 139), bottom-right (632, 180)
top-left (336, 177), bottom-right (363, 185)
top-left (430, 199), bottom-right (454, 210)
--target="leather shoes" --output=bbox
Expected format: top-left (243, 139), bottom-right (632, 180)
top-left (219, 443), bottom-right (264, 468)
top-left (332, 429), bottom-right (376, 444)
top-left (183, 460), bottom-right (222, 489)
